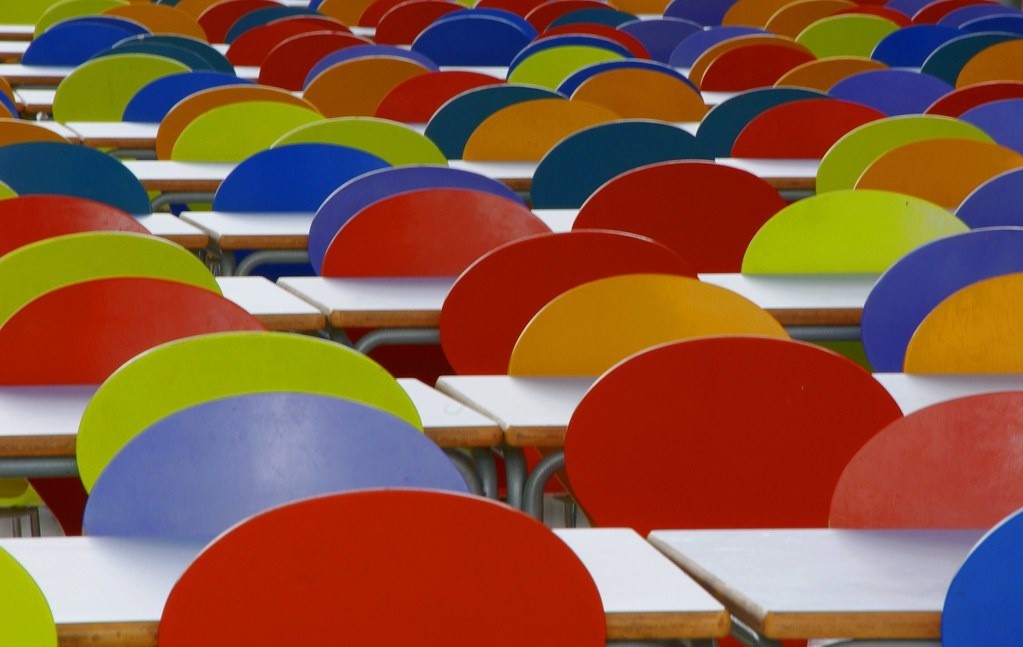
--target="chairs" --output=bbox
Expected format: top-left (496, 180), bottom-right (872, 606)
top-left (940, 507), bottom-right (1023, 646)
top-left (154, 488), bottom-right (609, 646)
top-left (0, 0), bottom-right (1023, 530)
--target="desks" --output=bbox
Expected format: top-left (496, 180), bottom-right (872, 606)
top-left (0, 28), bottom-right (1023, 646)
